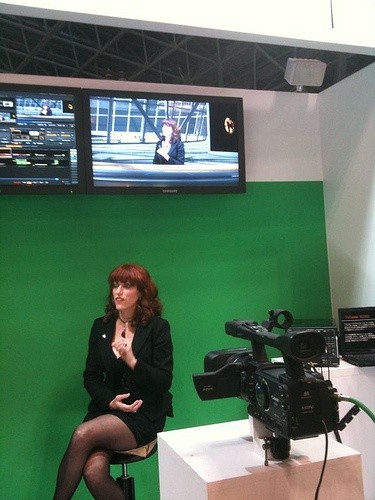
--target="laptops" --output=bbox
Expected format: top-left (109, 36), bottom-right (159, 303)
top-left (338, 307), bottom-right (375, 367)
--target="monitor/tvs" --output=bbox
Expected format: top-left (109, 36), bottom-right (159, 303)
top-left (82, 89), bottom-right (245, 194)
top-left (0, 82), bottom-right (87, 194)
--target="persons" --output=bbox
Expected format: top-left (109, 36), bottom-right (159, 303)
top-left (40, 104), bottom-right (52, 116)
top-left (153, 119), bottom-right (184, 164)
top-left (53, 263), bottom-right (174, 500)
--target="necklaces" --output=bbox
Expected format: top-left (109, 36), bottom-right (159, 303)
top-left (119, 317), bottom-right (130, 338)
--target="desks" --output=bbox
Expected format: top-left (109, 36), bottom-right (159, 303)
top-left (155, 419), bottom-right (366, 500)
top-left (270, 354), bottom-right (375, 500)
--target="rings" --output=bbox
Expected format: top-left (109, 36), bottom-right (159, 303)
top-left (123, 343), bottom-right (127, 346)
top-left (133, 411), bottom-right (136, 413)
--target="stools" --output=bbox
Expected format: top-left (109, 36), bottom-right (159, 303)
top-left (109, 442), bottom-right (158, 500)
